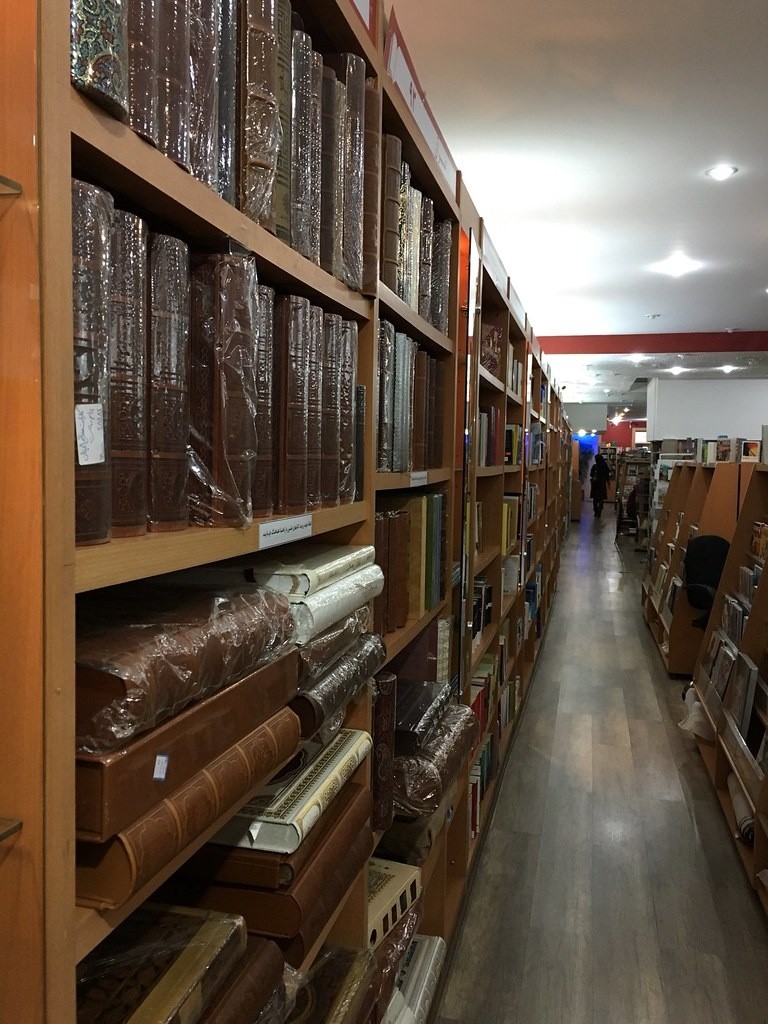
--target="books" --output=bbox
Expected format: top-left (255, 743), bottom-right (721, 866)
top-left (74, 544), bottom-right (450, 1024)
top-left (469, 342), bottom-right (566, 840)
top-left (622, 464), bottom-right (638, 518)
top-left (646, 509), bottom-right (698, 615)
top-left (71, 179), bottom-right (365, 544)
top-left (686, 424), bottom-right (768, 465)
top-left (699, 520), bottom-right (768, 783)
top-left (71, 0), bottom-right (379, 301)
top-left (377, 490), bottom-right (480, 822)
top-left (378, 133), bottom-right (451, 475)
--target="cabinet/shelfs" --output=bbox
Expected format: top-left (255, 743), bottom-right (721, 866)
top-left (598, 445), bottom-right (618, 503)
top-left (642, 462), bottom-right (737, 670)
top-left (675, 464), bottom-right (768, 916)
top-left (646, 438), bottom-right (695, 570)
top-left (0, 0), bottom-right (572, 1024)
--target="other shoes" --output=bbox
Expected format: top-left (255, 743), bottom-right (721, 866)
top-left (594, 513), bottom-right (599, 518)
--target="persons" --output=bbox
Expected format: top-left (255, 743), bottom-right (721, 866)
top-left (590, 454), bottom-right (611, 519)
